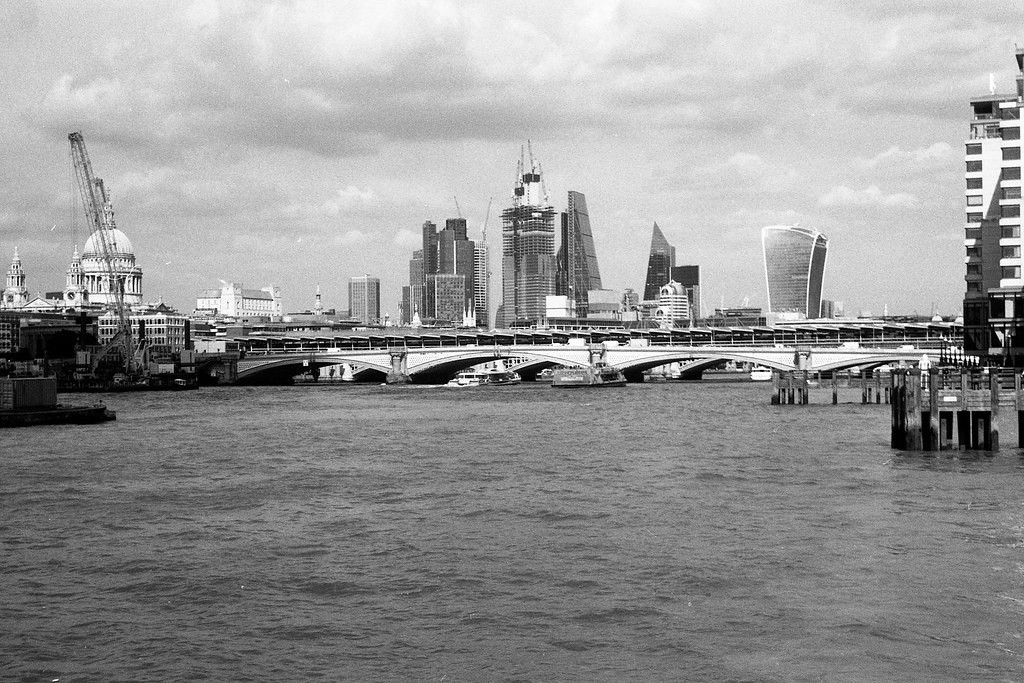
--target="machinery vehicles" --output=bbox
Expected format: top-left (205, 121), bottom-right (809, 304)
top-left (59, 129), bottom-right (176, 384)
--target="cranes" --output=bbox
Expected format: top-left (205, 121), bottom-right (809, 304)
top-left (513, 137), bottom-right (552, 208)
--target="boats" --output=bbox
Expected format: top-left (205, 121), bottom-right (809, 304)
top-left (0, 374), bottom-right (116, 426)
top-left (751, 365), bottom-right (773, 382)
top-left (446, 328), bottom-right (629, 388)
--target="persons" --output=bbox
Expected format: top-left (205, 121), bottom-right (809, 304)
top-left (971, 363), bottom-right (979, 390)
top-left (918, 354), bottom-right (932, 389)
